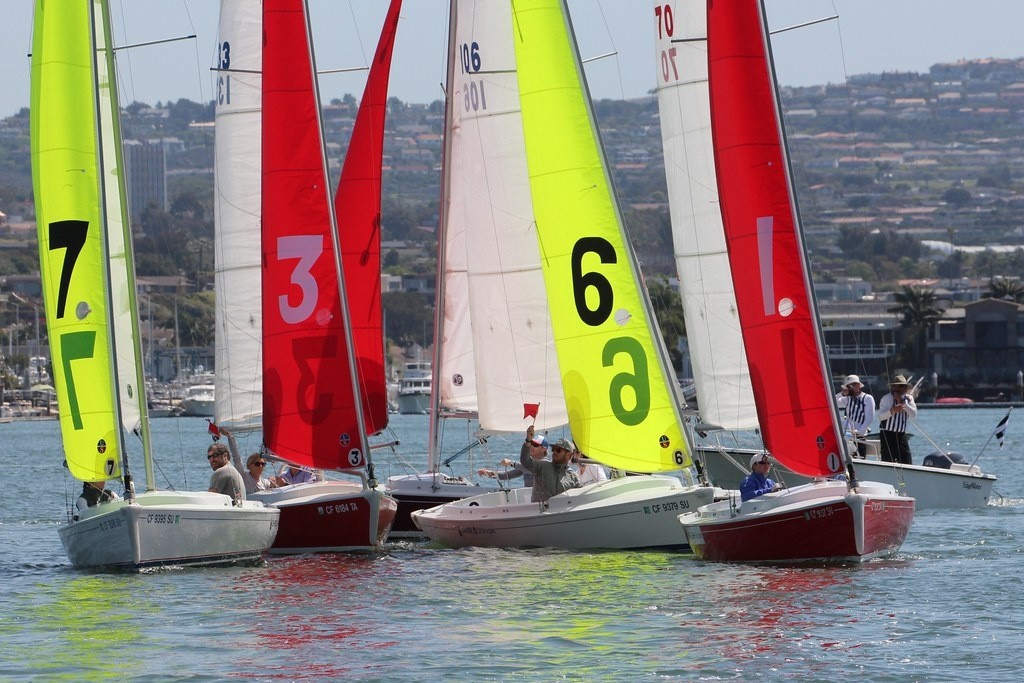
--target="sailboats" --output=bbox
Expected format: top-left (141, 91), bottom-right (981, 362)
top-left (376, 0), bottom-right (716, 549)
top-left (27, 0), bottom-right (285, 569)
top-left (212, 0), bottom-right (399, 552)
top-left (655, 0), bottom-right (916, 566)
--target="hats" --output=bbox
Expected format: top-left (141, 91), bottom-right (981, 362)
top-left (532, 435), bottom-right (548, 450)
top-left (887, 375), bottom-right (913, 392)
top-left (549, 438), bottom-right (573, 453)
top-left (750, 453), bottom-right (768, 468)
top-left (841, 375), bottom-right (864, 389)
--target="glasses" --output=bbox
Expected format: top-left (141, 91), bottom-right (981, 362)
top-left (531, 443), bottom-right (539, 447)
top-left (758, 461), bottom-right (771, 465)
top-left (846, 383), bottom-right (855, 388)
top-left (251, 462), bottom-right (266, 467)
top-left (207, 451), bottom-right (226, 460)
top-left (552, 448), bottom-right (562, 453)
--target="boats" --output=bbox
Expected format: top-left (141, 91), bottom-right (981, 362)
top-left (183, 381), bottom-right (216, 416)
top-left (698, 441), bottom-right (997, 510)
top-left (399, 361), bottom-right (433, 415)
top-left (150, 405), bottom-right (186, 417)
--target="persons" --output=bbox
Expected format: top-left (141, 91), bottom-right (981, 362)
top-left (220, 426), bottom-right (277, 494)
top-left (275, 462), bottom-right (316, 486)
top-left (738, 453), bottom-right (781, 503)
top-left (76, 481), bottom-right (114, 511)
top-left (519, 426), bottom-right (581, 502)
top-left (877, 374), bottom-right (918, 464)
top-left (569, 450), bottom-right (606, 486)
top-left (206, 443), bottom-right (247, 500)
top-left (477, 435), bottom-right (550, 488)
top-left (835, 375), bottom-right (875, 460)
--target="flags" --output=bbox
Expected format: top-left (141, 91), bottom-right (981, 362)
top-left (524, 403), bottom-right (538, 419)
top-left (208, 424), bottom-right (220, 438)
top-left (994, 414), bottom-right (1009, 450)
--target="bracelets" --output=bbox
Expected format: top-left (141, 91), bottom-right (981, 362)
top-left (510, 460), bottom-right (514, 467)
top-left (525, 438), bottom-right (532, 443)
